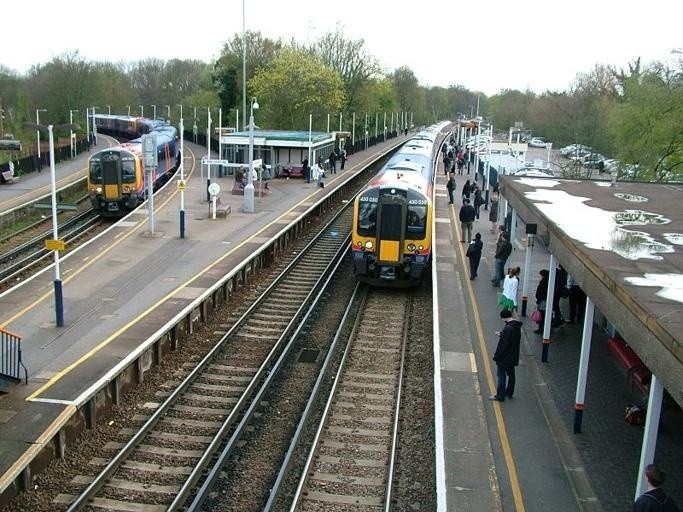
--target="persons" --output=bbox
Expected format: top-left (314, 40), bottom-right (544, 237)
top-left (631, 462), bottom-right (681, 511)
top-left (284, 143), bottom-right (349, 188)
top-left (440, 131), bottom-right (592, 335)
top-left (232, 163), bottom-right (272, 192)
top-left (486, 310), bottom-right (524, 401)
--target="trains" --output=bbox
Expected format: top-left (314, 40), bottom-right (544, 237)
top-left (87, 114), bottom-right (179, 218)
top-left (350, 119), bottom-right (453, 292)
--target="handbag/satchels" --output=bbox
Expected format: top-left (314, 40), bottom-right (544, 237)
top-left (563, 287), bottom-right (569, 300)
top-left (625, 405), bottom-right (645, 426)
top-left (466, 244), bottom-right (481, 257)
top-left (531, 311), bottom-right (542, 321)
top-left (497, 293), bottom-right (503, 304)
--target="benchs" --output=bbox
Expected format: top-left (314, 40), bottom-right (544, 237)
top-left (279, 166), bottom-right (304, 177)
top-left (231, 180), bottom-right (267, 197)
top-left (208, 197), bottom-right (231, 218)
top-left (1, 171), bottom-right (19, 185)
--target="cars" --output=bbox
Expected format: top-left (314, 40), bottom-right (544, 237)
top-left (499, 128), bottom-right (545, 149)
top-left (466, 115), bottom-right (491, 153)
top-left (558, 142), bottom-right (639, 179)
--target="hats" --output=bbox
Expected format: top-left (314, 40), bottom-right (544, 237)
top-left (500, 310), bottom-right (512, 318)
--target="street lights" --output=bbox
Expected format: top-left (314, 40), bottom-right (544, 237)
top-left (175, 103), bottom-right (183, 118)
top-left (187, 104), bottom-right (198, 143)
top-left (69, 109), bottom-right (79, 144)
top-left (124, 105), bottom-right (131, 116)
top-left (104, 105), bottom-right (111, 114)
top-left (163, 103), bottom-right (171, 118)
top-left (243, 96), bottom-right (260, 213)
top-left (36, 108), bottom-right (48, 158)
top-left (148, 104), bottom-right (157, 119)
top-left (138, 104), bottom-right (144, 117)
top-left (86, 107), bottom-right (94, 141)
top-left (92, 105), bottom-right (101, 114)
